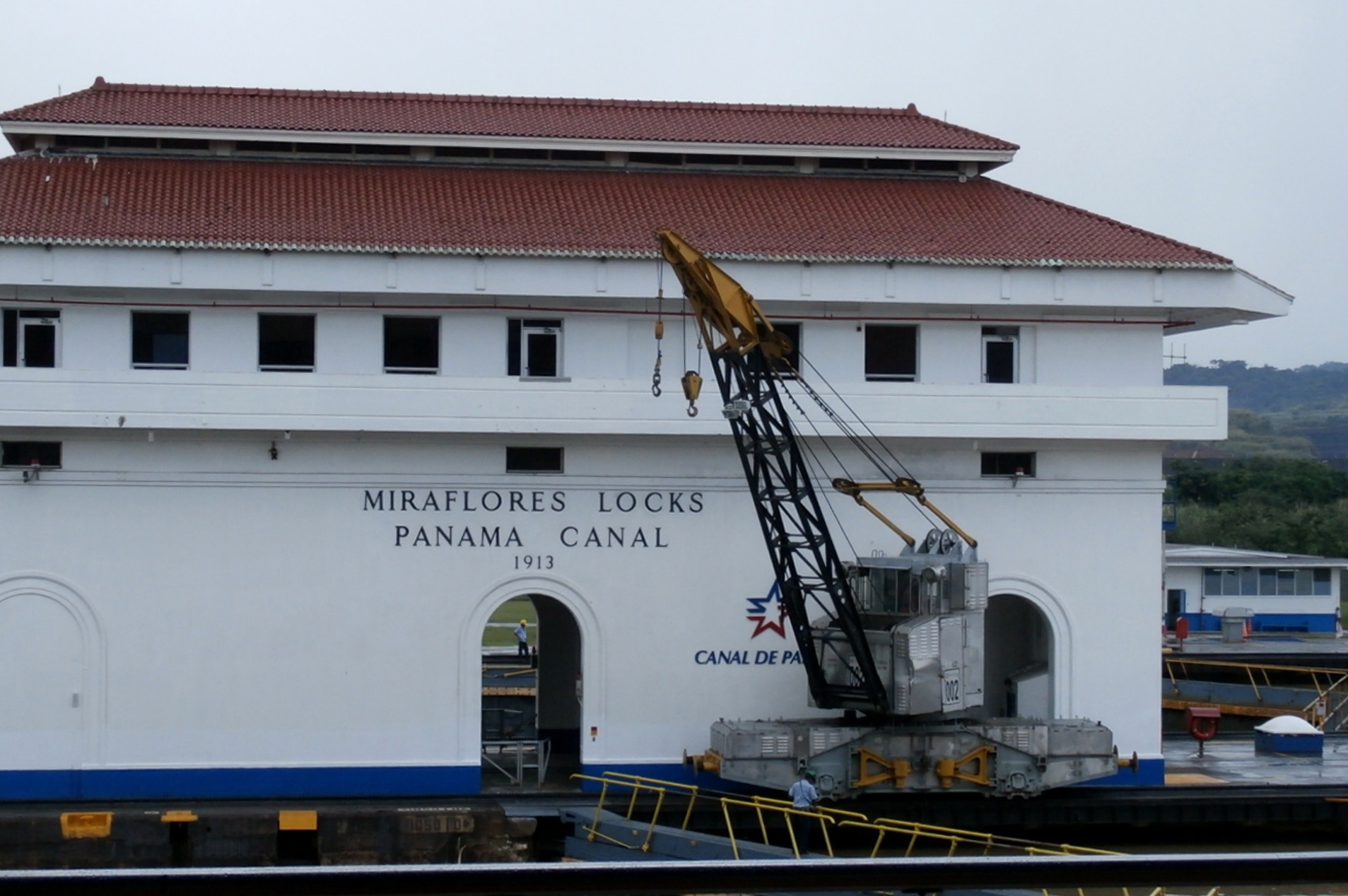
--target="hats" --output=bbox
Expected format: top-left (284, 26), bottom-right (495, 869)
top-left (806, 770), bottom-right (817, 784)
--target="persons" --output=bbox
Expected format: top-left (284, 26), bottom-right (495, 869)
top-left (789, 773), bottom-right (819, 855)
top-left (514, 619), bottom-right (528, 655)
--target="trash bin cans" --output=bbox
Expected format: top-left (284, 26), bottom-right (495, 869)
top-left (1177, 617), bottom-right (1187, 652)
top-left (278, 810), bottom-right (320, 866)
top-left (60, 811), bottom-right (112, 870)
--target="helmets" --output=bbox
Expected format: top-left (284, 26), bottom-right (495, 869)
top-left (520, 620), bottom-right (527, 625)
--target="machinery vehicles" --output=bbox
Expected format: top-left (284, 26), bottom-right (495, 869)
top-left (648, 225), bottom-right (1139, 797)
